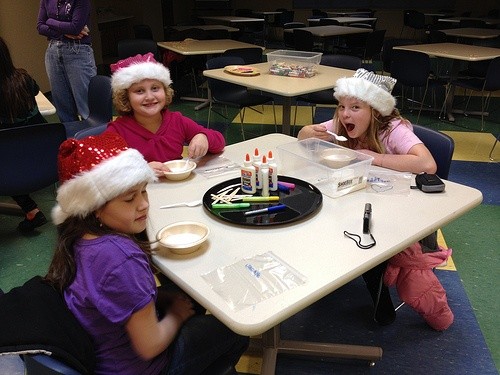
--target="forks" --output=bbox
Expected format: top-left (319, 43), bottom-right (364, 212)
top-left (159, 200), bottom-right (202, 208)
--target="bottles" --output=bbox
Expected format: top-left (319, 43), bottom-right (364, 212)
top-left (241, 148), bottom-right (278, 197)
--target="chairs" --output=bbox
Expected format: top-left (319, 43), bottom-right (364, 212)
top-left (0, 8), bottom-right (500, 375)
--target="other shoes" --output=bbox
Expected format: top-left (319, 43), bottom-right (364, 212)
top-left (374, 293), bottom-right (396, 325)
top-left (18, 211), bottom-right (48, 232)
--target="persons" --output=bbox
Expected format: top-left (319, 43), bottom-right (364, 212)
top-left (36, 0), bottom-right (96, 123)
top-left (297, 67), bottom-right (437, 325)
top-left (0, 36), bottom-right (56, 231)
top-left (44, 131), bottom-right (249, 375)
top-left (102, 52), bottom-right (225, 178)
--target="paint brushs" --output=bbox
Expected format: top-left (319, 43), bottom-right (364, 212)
top-left (212, 202), bottom-right (251, 208)
top-left (277, 181), bottom-right (295, 188)
top-left (278, 184), bottom-right (290, 192)
top-left (243, 196), bottom-right (280, 201)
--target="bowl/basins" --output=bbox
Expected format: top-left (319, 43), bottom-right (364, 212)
top-left (155, 221), bottom-right (210, 254)
top-left (162, 160), bottom-right (197, 181)
top-left (317, 147), bottom-right (359, 169)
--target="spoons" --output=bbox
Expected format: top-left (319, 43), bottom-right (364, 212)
top-left (325, 130), bottom-right (348, 142)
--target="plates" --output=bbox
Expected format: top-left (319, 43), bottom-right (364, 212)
top-left (224, 65), bottom-right (259, 76)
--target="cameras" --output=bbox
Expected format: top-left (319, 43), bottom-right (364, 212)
top-left (363, 203), bottom-right (371, 233)
top-left (416, 174), bottom-right (445, 192)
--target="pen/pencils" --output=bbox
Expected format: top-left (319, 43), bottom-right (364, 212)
top-left (244, 204), bottom-right (287, 217)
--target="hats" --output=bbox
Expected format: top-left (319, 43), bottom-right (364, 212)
top-left (50, 133), bottom-right (159, 226)
top-left (333, 68), bottom-right (398, 116)
top-left (110, 52), bottom-right (173, 92)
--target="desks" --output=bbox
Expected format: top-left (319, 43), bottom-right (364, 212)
top-left (438, 17), bottom-right (500, 29)
top-left (424, 13), bottom-right (459, 18)
top-left (198, 16), bottom-right (264, 41)
top-left (284, 25), bottom-right (373, 55)
top-left (326, 11), bottom-right (373, 17)
top-left (437, 27), bottom-right (500, 46)
top-left (157, 39), bottom-right (265, 110)
top-left (254, 11), bottom-right (283, 48)
top-left (146, 133), bottom-right (483, 375)
top-left (171, 25), bottom-right (240, 40)
top-left (393, 43), bottom-right (500, 118)
top-left (307, 17), bottom-right (378, 27)
